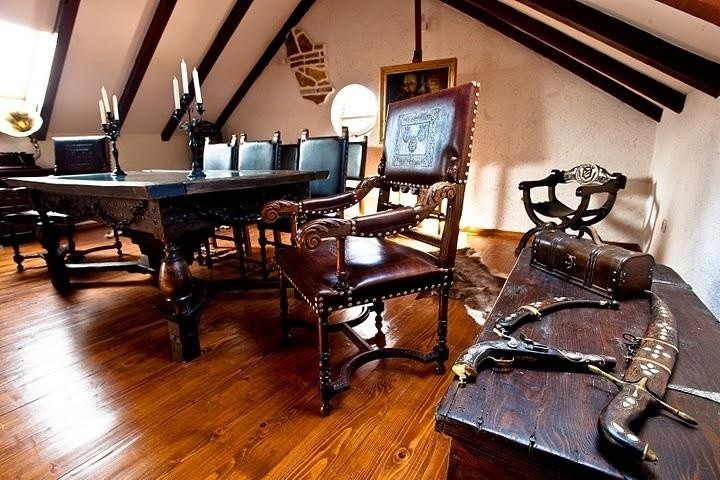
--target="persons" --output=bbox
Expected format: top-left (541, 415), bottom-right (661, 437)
top-left (389, 73), bottom-right (429, 100)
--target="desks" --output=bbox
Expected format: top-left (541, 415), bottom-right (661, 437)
top-left (5, 168), bottom-right (330, 363)
top-left (435, 263), bottom-right (720, 480)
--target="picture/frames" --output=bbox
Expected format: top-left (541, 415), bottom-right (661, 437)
top-left (378, 57), bottom-right (457, 145)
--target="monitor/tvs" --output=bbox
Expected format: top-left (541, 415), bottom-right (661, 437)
top-left (53, 136), bottom-right (112, 173)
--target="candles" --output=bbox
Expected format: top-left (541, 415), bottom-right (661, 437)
top-left (99, 86), bottom-right (120, 124)
top-left (173, 57), bottom-right (202, 109)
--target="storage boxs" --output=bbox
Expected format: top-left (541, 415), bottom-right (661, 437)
top-left (529, 228), bottom-right (655, 301)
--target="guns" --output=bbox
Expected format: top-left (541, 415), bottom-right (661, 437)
top-left (452, 334), bottom-right (616, 387)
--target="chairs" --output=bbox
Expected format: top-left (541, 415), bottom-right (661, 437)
top-left (517, 162), bottom-right (627, 250)
top-left (195, 127), bottom-right (368, 286)
top-left (0, 135), bottom-right (123, 272)
top-left (258, 80), bottom-right (479, 417)
